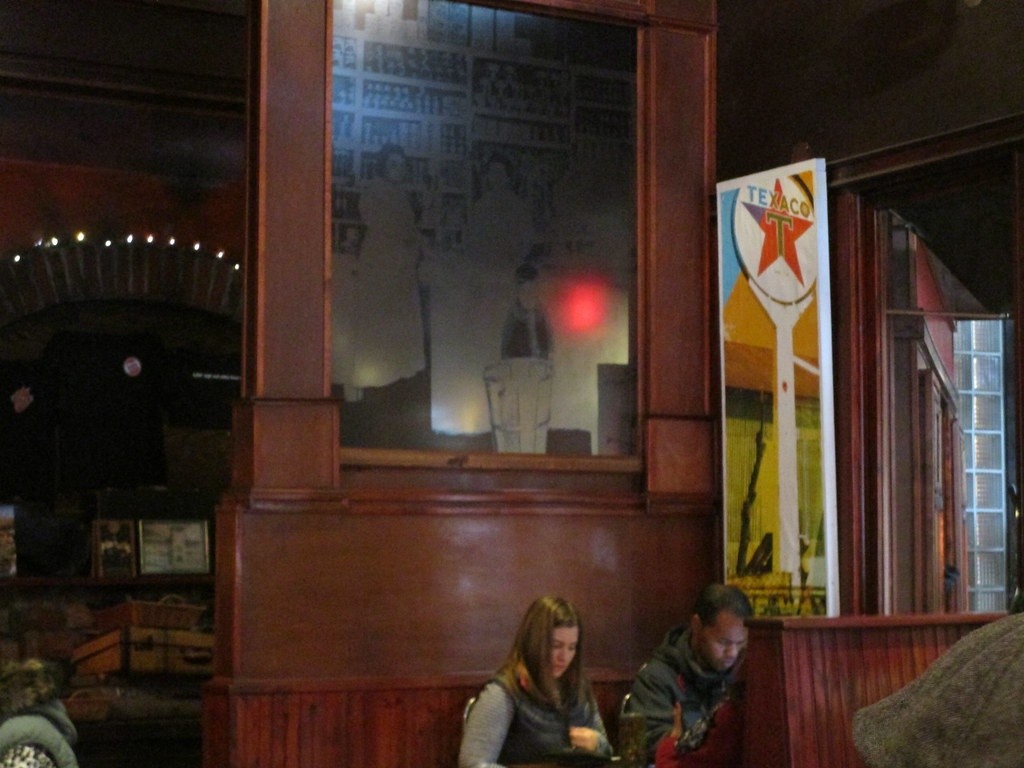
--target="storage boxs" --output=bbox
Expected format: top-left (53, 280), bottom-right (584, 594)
top-left (69, 624), bottom-right (214, 678)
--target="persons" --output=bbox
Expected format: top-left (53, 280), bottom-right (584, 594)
top-left (459, 597), bottom-right (613, 768)
top-left (630, 585), bottom-right (755, 767)
top-left (0, 659), bottom-right (78, 768)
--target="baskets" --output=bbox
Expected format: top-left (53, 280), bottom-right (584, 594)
top-left (65, 687), bottom-right (180, 725)
top-left (95, 595), bottom-right (208, 635)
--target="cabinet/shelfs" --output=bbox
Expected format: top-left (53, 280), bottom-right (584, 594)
top-left (331, 32), bottom-right (636, 277)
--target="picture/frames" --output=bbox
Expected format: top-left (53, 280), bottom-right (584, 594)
top-left (92, 518), bottom-right (211, 576)
